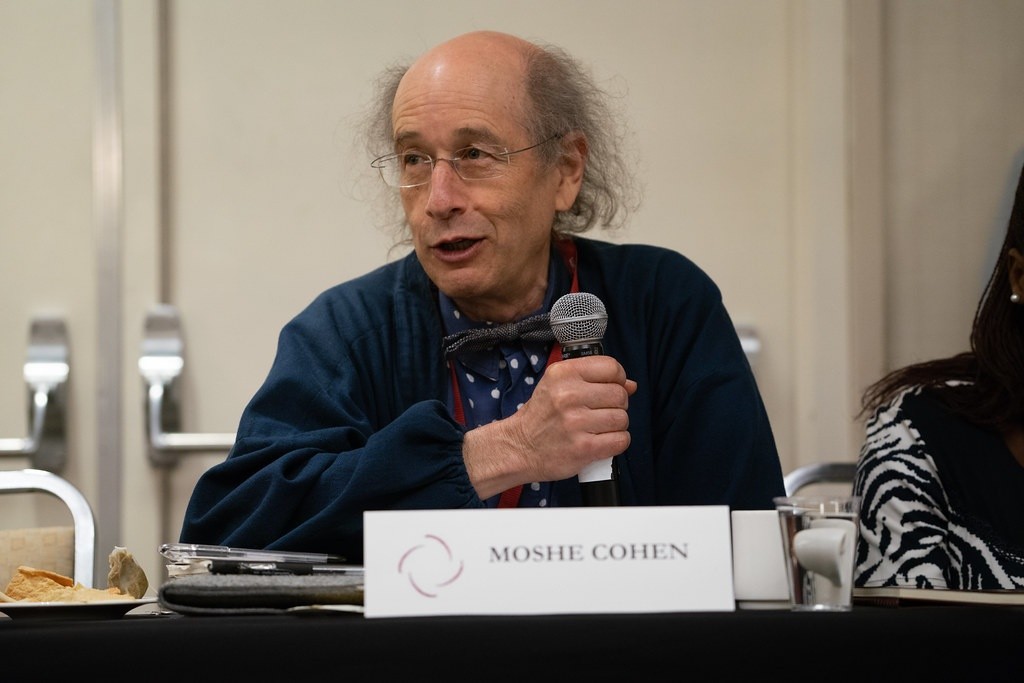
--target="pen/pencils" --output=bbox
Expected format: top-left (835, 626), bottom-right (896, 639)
top-left (156, 541), bottom-right (363, 574)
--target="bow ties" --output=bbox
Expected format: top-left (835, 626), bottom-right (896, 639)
top-left (442, 312), bottom-right (555, 362)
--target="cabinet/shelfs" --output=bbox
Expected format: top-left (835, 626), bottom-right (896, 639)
top-left (1, 1), bottom-right (849, 603)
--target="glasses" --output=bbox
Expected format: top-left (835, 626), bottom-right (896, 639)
top-left (371, 134), bottom-right (557, 188)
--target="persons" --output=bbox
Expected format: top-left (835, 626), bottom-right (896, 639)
top-left (849, 158), bottom-right (1024, 593)
top-left (179, 31), bottom-right (786, 567)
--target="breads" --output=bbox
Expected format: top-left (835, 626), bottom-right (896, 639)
top-left (106, 546), bottom-right (148, 599)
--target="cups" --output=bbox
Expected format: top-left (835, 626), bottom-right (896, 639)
top-left (773, 495), bottom-right (862, 611)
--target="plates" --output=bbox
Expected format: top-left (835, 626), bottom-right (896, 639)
top-left (0, 597), bottom-right (159, 619)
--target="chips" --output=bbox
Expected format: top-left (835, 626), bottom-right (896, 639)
top-left (4, 565), bottom-right (136, 602)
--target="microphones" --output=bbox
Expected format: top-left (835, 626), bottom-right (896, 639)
top-left (550, 292), bottom-right (619, 508)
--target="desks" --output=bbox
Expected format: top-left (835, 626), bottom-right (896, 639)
top-left (0, 607), bottom-right (1023, 683)
